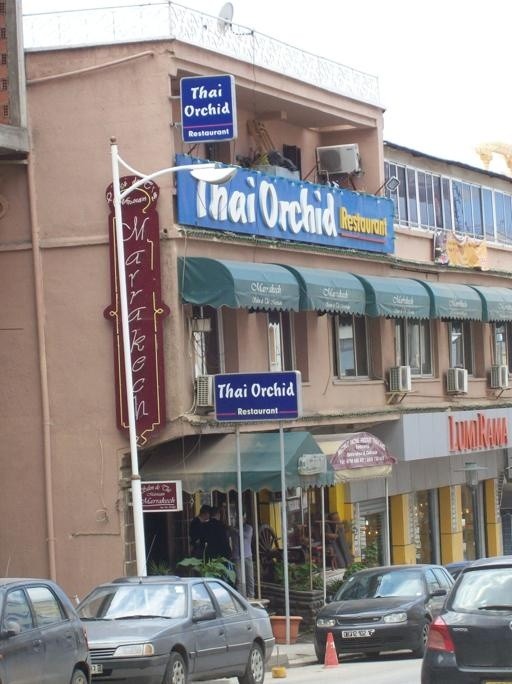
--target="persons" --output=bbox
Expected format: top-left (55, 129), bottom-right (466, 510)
top-left (190, 503), bottom-right (210, 578)
top-left (202, 507), bottom-right (232, 583)
top-left (230, 510), bottom-right (254, 599)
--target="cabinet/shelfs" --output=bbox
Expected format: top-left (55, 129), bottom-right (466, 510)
top-left (255, 165), bottom-right (300, 180)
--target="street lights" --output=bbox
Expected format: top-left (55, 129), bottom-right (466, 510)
top-left (108, 135), bottom-right (237, 575)
top-left (454, 462), bottom-right (490, 559)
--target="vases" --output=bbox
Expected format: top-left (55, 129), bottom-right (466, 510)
top-left (270, 616), bottom-right (303, 643)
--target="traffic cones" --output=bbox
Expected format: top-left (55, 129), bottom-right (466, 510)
top-left (322, 632), bottom-right (341, 668)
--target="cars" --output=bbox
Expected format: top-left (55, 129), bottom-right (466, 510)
top-left (445, 561), bottom-right (473, 580)
top-left (74, 575), bottom-right (276, 684)
top-left (0, 578), bottom-right (92, 684)
top-left (422, 555), bottom-right (512, 684)
top-left (314, 564), bottom-right (455, 663)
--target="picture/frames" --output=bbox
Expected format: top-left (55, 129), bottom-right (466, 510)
top-left (269, 326), bottom-right (276, 364)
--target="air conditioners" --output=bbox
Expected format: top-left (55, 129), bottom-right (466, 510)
top-left (316, 143), bottom-right (362, 175)
top-left (491, 365), bottom-right (509, 388)
top-left (197, 375), bottom-right (212, 407)
top-left (447, 368), bottom-right (468, 393)
top-left (390, 366), bottom-right (412, 392)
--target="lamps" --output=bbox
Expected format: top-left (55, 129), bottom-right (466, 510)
top-left (451, 328), bottom-right (462, 344)
top-left (374, 175), bottom-right (401, 196)
